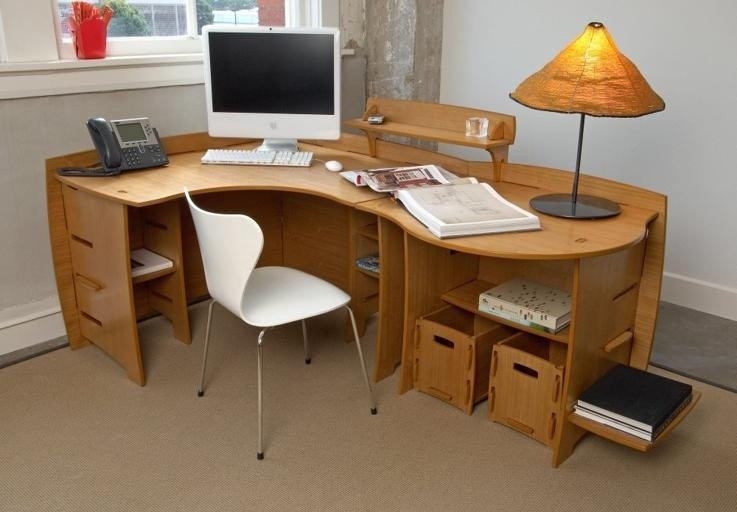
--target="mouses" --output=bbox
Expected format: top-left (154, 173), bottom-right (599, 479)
top-left (323, 158), bottom-right (344, 173)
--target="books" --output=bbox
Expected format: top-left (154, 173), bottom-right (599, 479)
top-left (476, 277), bottom-right (573, 336)
top-left (339, 164), bottom-right (541, 239)
top-left (573, 363), bottom-right (693, 442)
top-left (130, 248), bottom-right (175, 279)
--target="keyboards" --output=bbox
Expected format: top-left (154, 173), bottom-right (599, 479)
top-left (201, 146), bottom-right (315, 168)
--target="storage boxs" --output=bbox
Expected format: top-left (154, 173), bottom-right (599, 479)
top-left (486, 331), bottom-right (566, 449)
top-left (413, 304), bottom-right (512, 415)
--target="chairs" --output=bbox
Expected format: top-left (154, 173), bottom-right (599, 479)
top-left (183, 187), bottom-right (379, 460)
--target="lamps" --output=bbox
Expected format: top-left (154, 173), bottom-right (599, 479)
top-left (508, 21), bottom-right (666, 220)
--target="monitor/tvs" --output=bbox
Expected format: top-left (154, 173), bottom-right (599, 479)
top-left (203, 24), bottom-right (342, 151)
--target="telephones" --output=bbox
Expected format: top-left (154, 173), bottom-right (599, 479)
top-left (83, 118), bottom-right (170, 177)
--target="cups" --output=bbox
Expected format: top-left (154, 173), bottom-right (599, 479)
top-left (465, 117), bottom-right (489, 138)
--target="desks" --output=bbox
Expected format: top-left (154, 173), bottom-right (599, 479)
top-left (354, 161), bottom-right (701, 470)
top-left (44, 132), bottom-right (468, 469)
